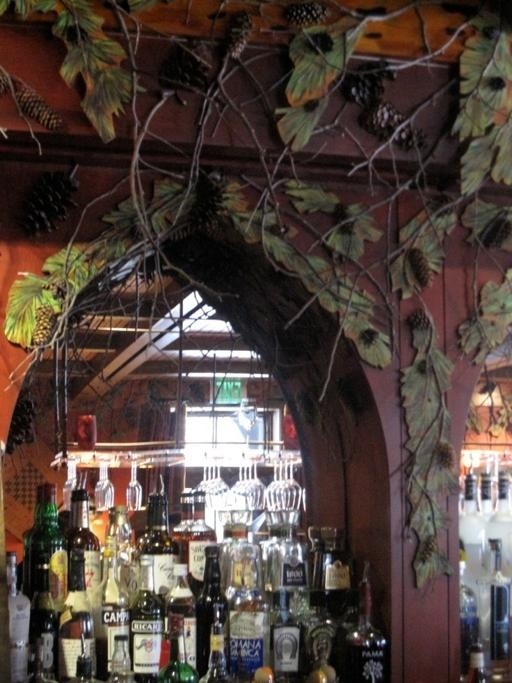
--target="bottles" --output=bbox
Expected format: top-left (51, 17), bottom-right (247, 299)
top-left (455, 472), bottom-right (512, 683)
top-left (5, 482), bottom-right (386, 681)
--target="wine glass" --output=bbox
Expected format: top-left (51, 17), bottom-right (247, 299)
top-left (237, 397), bottom-right (258, 452)
top-left (61, 453), bottom-right (301, 513)
